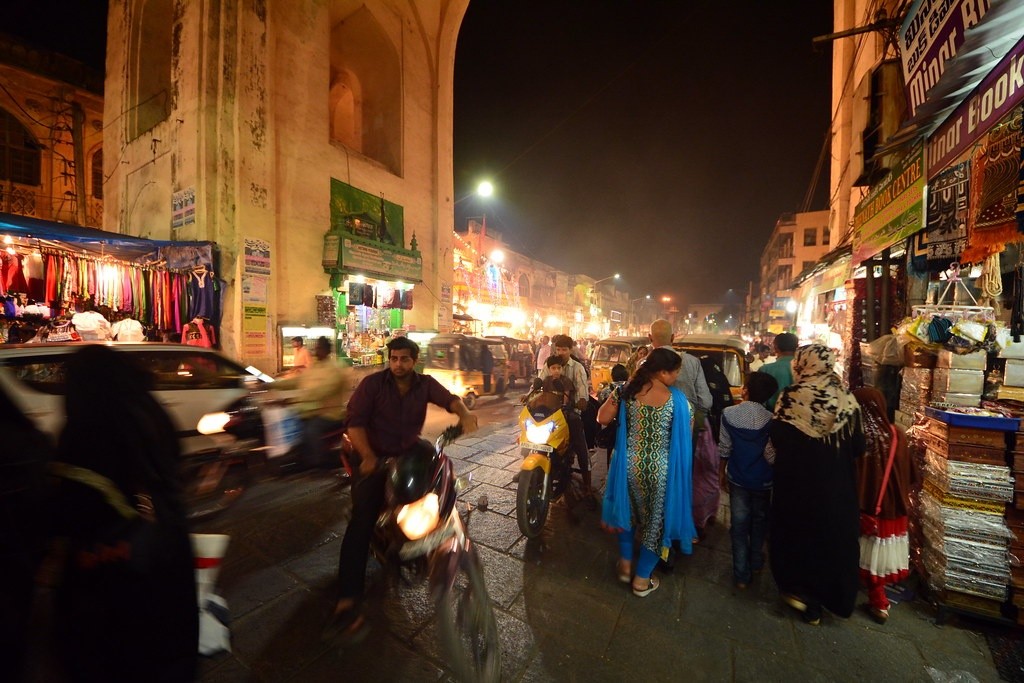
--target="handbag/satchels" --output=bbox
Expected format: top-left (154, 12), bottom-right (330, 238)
top-left (861, 512), bottom-right (878, 536)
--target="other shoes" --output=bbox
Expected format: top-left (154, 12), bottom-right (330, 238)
top-left (332, 600), bottom-right (369, 630)
top-left (783, 589), bottom-right (806, 608)
top-left (583, 495), bottom-right (598, 512)
top-left (737, 574), bottom-right (749, 588)
top-left (752, 551), bottom-right (765, 574)
top-left (868, 603), bottom-right (887, 623)
top-left (802, 604), bottom-right (823, 626)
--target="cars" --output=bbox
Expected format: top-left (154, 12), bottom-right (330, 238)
top-left (0, 342), bottom-right (276, 505)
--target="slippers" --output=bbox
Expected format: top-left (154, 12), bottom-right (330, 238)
top-left (614, 560), bottom-right (632, 585)
top-left (634, 574), bottom-right (660, 597)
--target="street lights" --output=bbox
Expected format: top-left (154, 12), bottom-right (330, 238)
top-left (631, 295), bottom-right (651, 328)
top-left (594, 274), bottom-right (621, 335)
top-left (475, 179), bottom-right (494, 311)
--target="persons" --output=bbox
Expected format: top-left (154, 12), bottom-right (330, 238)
top-left (0, 344), bottom-right (199, 682)
top-left (334, 336), bottom-right (479, 631)
top-left (254, 336), bottom-right (355, 456)
top-left (519, 318), bottom-right (924, 624)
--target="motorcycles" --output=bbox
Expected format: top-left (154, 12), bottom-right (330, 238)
top-left (423, 332), bottom-right (512, 411)
top-left (485, 335), bottom-right (537, 391)
top-left (517, 379), bottom-right (599, 540)
top-left (672, 332), bottom-right (756, 405)
top-left (336, 419), bottom-right (502, 683)
top-left (583, 336), bottom-right (652, 397)
top-left (175, 371), bottom-right (357, 525)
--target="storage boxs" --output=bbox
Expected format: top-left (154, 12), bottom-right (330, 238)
top-left (932, 329), bottom-right (1024, 407)
